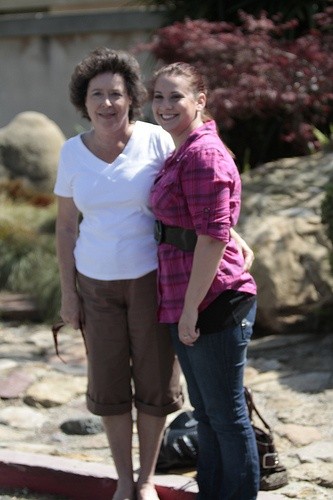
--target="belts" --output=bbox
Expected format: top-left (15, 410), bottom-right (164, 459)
top-left (152, 219), bottom-right (197, 252)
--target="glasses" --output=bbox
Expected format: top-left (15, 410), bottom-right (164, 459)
top-left (51, 318), bottom-right (88, 364)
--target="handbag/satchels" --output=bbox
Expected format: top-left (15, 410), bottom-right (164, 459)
top-left (157, 410), bottom-right (199, 471)
top-left (243, 386), bottom-right (289, 490)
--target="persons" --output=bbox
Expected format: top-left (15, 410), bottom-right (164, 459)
top-left (53, 45), bottom-right (186, 500)
top-left (146, 62), bottom-right (260, 500)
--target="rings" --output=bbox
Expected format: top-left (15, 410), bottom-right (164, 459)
top-left (183, 335), bottom-right (190, 340)
